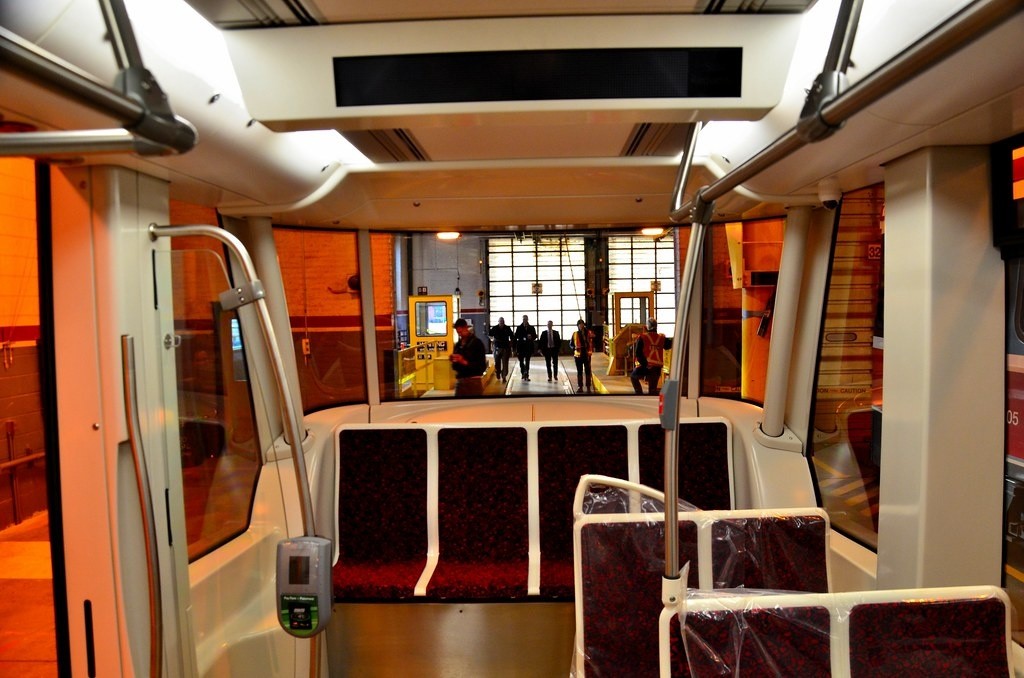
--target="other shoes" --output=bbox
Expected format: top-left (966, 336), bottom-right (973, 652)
top-left (576, 388), bottom-right (583, 393)
top-left (496, 372), bottom-right (500, 379)
top-left (554, 376), bottom-right (558, 380)
top-left (525, 377), bottom-right (530, 381)
top-left (548, 378), bottom-right (552, 381)
top-left (587, 386), bottom-right (591, 393)
top-left (503, 376), bottom-right (506, 382)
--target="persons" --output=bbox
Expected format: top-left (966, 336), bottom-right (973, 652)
top-left (488, 317), bottom-right (515, 382)
top-left (569, 320), bottom-right (595, 392)
top-left (537, 321), bottom-right (561, 381)
top-left (450, 318), bottom-right (487, 396)
top-left (630, 318), bottom-right (672, 394)
top-left (515, 315), bottom-right (537, 380)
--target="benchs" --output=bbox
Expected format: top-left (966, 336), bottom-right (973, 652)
top-left (329, 416), bottom-right (734, 602)
top-left (659, 583), bottom-right (1017, 678)
top-left (574, 508), bottom-right (832, 678)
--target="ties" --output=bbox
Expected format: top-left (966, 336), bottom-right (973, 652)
top-left (549, 331), bottom-right (552, 346)
